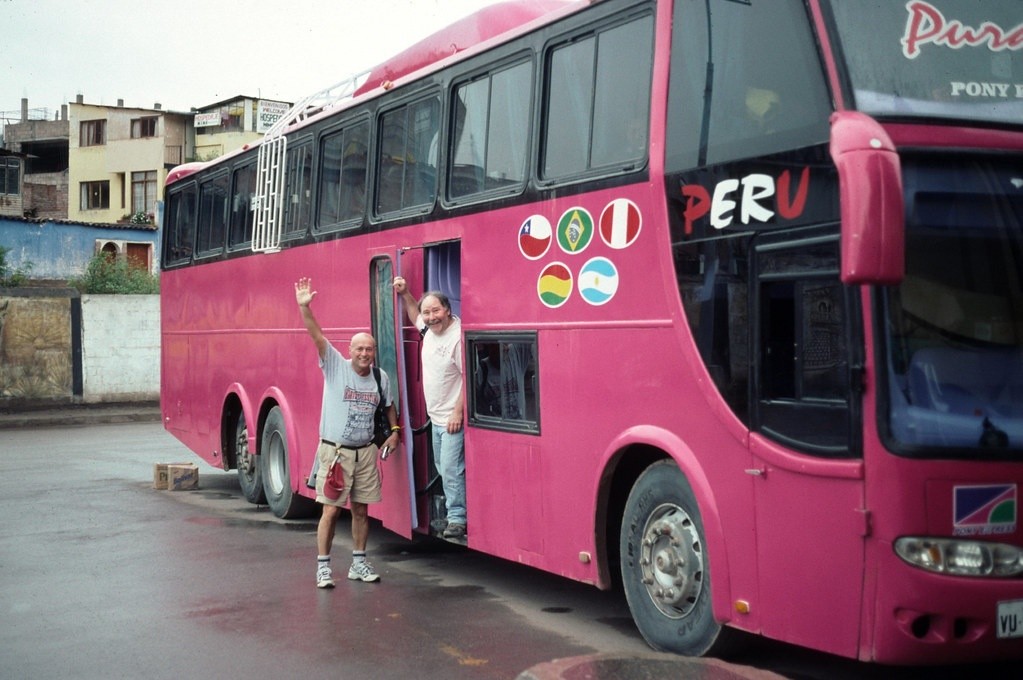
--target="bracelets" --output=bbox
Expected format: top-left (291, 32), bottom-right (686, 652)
top-left (391, 426), bottom-right (400, 433)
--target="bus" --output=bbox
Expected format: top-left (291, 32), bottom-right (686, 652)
top-left (159, 0), bottom-right (1023, 671)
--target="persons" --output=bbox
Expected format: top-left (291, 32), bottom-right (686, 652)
top-left (394, 275), bottom-right (467, 537)
top-left (293, 277), bottom-right (400, 589)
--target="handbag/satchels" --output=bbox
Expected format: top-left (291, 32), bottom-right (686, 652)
top-left (374, 403), bottom-right (392, 449)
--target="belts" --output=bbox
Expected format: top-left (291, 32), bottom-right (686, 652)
top-left (322, 439), bottom-right (375, 451)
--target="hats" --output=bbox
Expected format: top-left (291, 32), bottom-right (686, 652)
top-left (322, 463), bottom-right (345, 500)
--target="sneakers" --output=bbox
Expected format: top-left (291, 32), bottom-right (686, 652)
top-left (348, 558), bottom-right (381, 582)
top-left (315, 565), bottom-right (336, 588)
top-left (443, 523), bottom-right (466, 537)
top-left (430, 518), bottom-right (448, 532)
